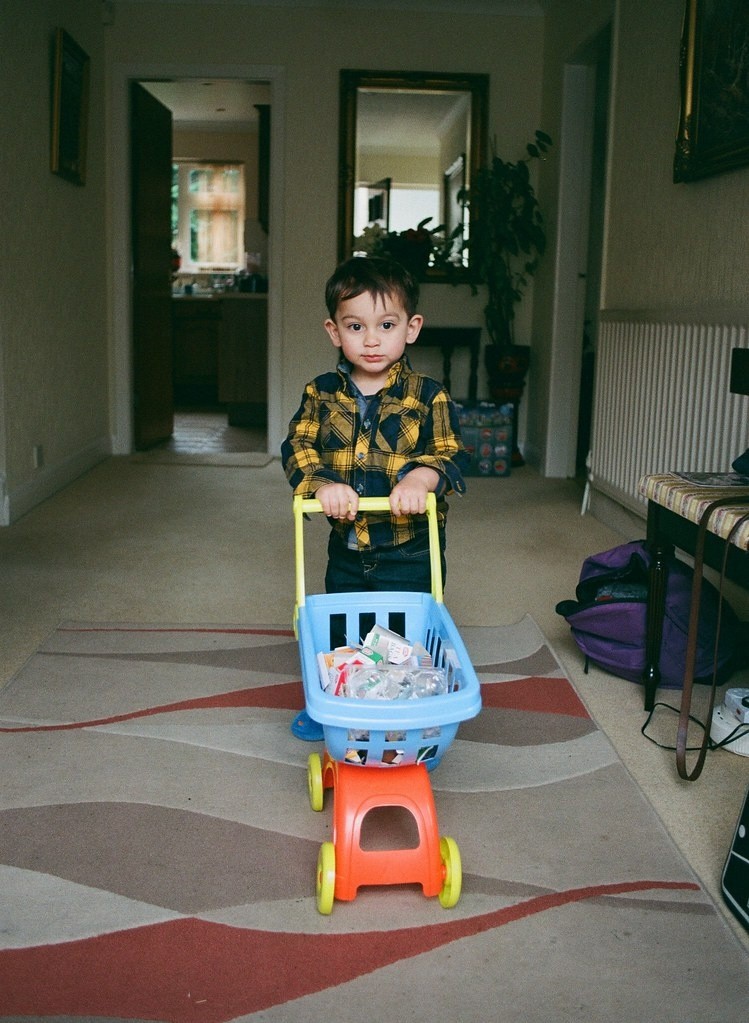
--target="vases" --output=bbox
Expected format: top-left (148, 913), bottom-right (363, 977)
top-left (391, 245), bottom-right (432, 277)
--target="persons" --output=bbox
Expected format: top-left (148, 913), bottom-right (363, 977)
top-left (280, 256), bottom-right (466, 741)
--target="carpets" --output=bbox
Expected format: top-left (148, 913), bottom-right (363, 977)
top-left (0, 614), bottom-right (749, 1022)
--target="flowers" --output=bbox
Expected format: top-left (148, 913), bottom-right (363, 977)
top-left (351, 217), bottom-right (465, 276)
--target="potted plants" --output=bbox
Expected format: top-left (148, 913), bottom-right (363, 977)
top-left (457, 131), bottom-right (553, 468)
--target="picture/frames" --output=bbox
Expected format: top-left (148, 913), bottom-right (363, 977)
top-left (672, 0), bottom-right (749, 183)
top-left (50, 27), bottom-right (92, 186)
top-left (442, 154), bottom-right (466, 237)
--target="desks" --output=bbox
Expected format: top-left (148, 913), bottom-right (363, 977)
top-left (342, 326), bottom-right (483, 400)
top-left (639, 472), bottom-right (749, 711)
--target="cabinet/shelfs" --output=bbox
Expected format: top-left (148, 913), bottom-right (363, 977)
top-left (175, 301), bottom-right (268, 429)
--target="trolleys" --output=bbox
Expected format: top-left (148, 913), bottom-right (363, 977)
top-left (294, 493), bottom-right (482, 918)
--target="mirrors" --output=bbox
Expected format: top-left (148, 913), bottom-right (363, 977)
top-left (338, 67), bottom-right (490, 284)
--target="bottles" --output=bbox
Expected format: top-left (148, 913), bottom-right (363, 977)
top-left (456, 404), bottom-right (463, 417)
top-left (479, 402), bottom-right (495, 423)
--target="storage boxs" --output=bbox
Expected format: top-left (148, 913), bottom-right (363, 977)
top-left (452, 397), bottom-right (514, 478)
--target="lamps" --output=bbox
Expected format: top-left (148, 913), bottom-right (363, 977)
top-left (731, 347), bottom-right (749, 474)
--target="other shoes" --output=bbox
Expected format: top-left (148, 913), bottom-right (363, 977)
top-left (292, 711), bottom-right (324, 743)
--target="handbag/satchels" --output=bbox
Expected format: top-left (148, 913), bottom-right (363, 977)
top-left (556, 538), bottom-right (744, 688)
